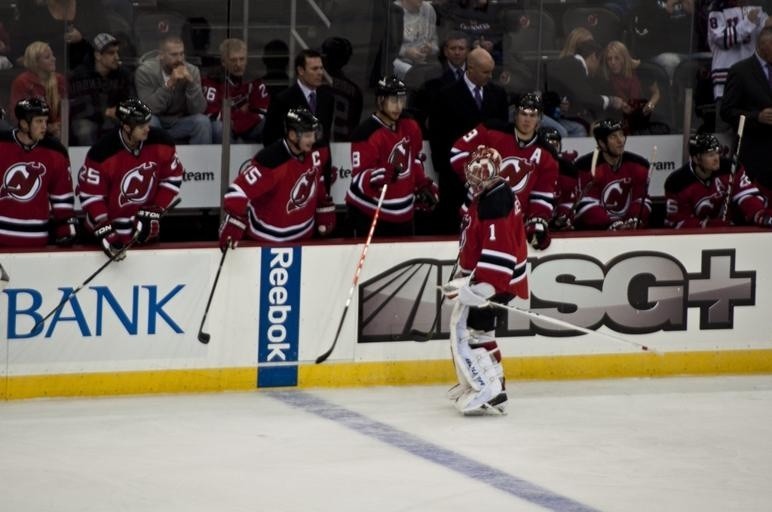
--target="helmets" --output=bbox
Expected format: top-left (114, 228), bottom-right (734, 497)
top-left (115, 96), bottom-right (153, 124)
top-left (374, 74), bottom-right (409, 97)
top-left (273, 105), bottom-right (320, 138)
top-left (591, 118), bottom-right (625, 141)
top-left (462, 143), bottom-right (504, 179)
top-left (15, 95), bottom-right (51, 122)
top-left (687, 132), bottom-right (720, 157)
top-left (537, 126), bottom-right (562, 147)
top-left (519, 94), bottom-right (545, 112)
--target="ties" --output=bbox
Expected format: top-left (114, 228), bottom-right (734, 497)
top-left (472, 86), bottom-right (483, 109)
top-left (455, 68), bottom-right (465, 81)
top-left (766, 65), bottom-right (772, 89)
top-left (308, 92), bottom-right (318, 113)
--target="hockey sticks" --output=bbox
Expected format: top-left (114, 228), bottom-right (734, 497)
top-left (316, 186), bottom-right (387, 363)
top-left (197, 242), bottom-right (231, 343)
top-left (29, 197), bottom-right (181, 336)
top-left (489, 299), bottom-right (667, 356)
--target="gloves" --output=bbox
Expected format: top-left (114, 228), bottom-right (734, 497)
top-left (710, 216), bottom-right (725, 227)
top-left (524, 217), bottom-right (551, 251)
top-left (217, 211), bottom-right (250, 253)
top-left (314, 204), bottom-right (337, 236)
top-left (131, 207), bottom-right (162, 245)
top-left (362, 167), bottom-right (397, 198)
top-left (93, 219), bottom-right (129, 262)
top-left (413, 178), bottom-right (440, 214)
top-left (752, 208), bottom-right (772, 226)
top-left (55, 220), bottom-right (79, 245)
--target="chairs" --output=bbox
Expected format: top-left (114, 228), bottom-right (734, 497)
top-left (494, 9), bottom-right (714, 135)
top-left (405, 65), bottom-right (442, 87)
top-left (119, 21), bottom-right (311, 86)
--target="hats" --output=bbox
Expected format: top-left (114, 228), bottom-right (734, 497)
top-left (93, 32), bottom-right (122, 52)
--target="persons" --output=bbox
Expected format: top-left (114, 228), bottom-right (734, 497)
top-left (440, 145), bottom-right (528, 417)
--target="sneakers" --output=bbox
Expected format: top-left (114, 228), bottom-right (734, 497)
top-left (445, 382), bottom-right (510, 412)
